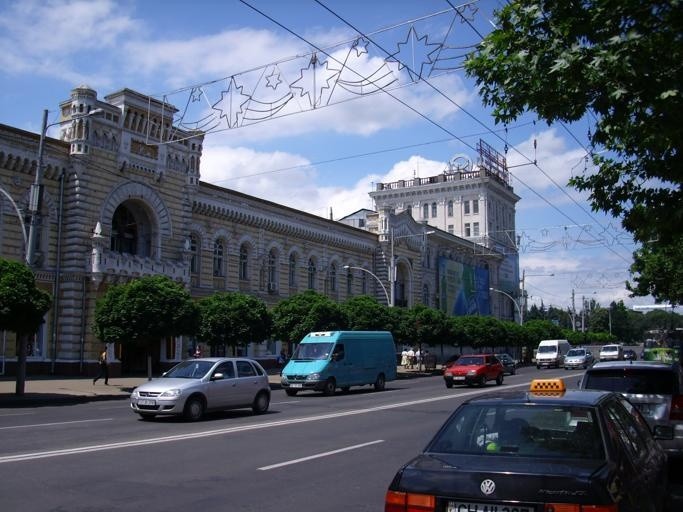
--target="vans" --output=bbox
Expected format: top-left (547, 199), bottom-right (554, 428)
top-left (535, 339), bottom-right (570, 369)
top-left (598, 343), bottom-right (622, 362)
top-left (576, 358), bottom-right (683, 461)
top-left (280, 328), bottom-right (398, 397)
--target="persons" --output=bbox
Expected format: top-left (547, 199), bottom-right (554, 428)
top-left (93, 346), bottom-right (109, 385)
top-left (276, 348), bottom-right (286, 377)
top-left (193, 345), bottom-right (203, 358)
top-left (401, 348), bottom-right (430, 370)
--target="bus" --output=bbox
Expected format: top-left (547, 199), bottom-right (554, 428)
top-left (641, 327), bottom-right (683, 361)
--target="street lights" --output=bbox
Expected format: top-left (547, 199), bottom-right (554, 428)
top-left (548, 288), bottom-right (597, 331)
top-left (0, 107), bottom-right (105, 401)
top-left (488, 268), bottom-right (555, 364)
top-left (343, 227), bottom-right (434, 309)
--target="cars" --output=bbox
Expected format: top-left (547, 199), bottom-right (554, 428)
top-left (563, 348), bottom-right (594, 369)
top-left (129, 357), bottom-right (270, 423)
top-left (384, 377), bottom-right (674, 512)
top-left (493, 353), bottom-right (515, 374)
top-left (444, 353), bottom-right (504, 389)
top-left (623, 350), bottom-right (636, 360)
